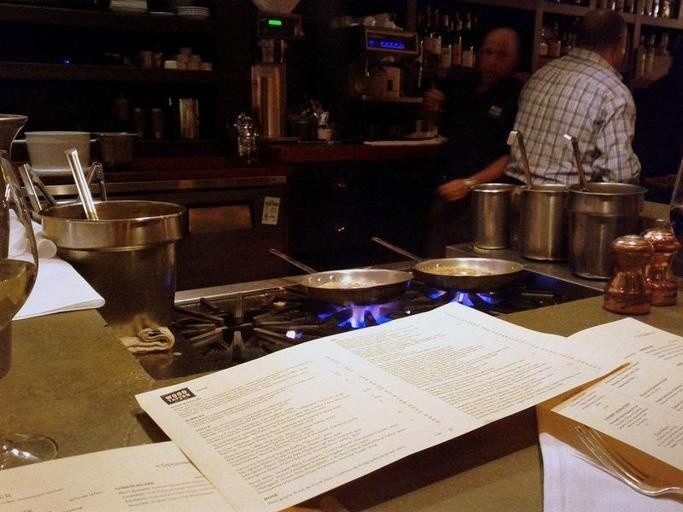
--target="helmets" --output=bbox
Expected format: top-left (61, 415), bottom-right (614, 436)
top-left (150, 6), bottom-right (209, 16)
top-left (30, 168), bottom-right (90, 178)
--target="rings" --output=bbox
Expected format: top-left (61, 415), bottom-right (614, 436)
top-left (428, 104), bottom-right (432, 108)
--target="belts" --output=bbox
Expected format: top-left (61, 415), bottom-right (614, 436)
top-left (0, 157), bottom-right (58, 469)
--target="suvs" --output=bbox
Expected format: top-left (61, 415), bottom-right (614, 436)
top-left (36, 198), bottom-right (188, 333)
top-left (24, 132), bottom-right (88, 172)
top-left (564, 183), bottom-right (648, 281)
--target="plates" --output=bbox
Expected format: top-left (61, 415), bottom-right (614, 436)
top-left (506, 1), bottom-right (682, 92)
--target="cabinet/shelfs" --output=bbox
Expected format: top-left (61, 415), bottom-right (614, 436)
top-left (539, 14), bottom-right (578, 58)
top-left (638, 31), bottom-right (672, 80)
top-left (604, 217), bottom-right (682, 316)
top-left (425, 3), bottom-right (474, 69)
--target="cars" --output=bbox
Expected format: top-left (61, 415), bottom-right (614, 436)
top-left (460, 180), bottom-right (648, 281)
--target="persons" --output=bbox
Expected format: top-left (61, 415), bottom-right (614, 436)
top-left (503, 8), bottom-right (641, 188)
top-left (422, 27), bottom-right (526, 201)
top-left (631, 31), bottom-right (683, 200)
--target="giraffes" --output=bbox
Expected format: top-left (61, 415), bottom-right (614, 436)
top-left (172, 261), bottom-right (603, 375)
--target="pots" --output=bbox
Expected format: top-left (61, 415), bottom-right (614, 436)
top-left (89, 131), bottom-right (138, 171)
top-left (35, 199), bottom-right (188, 336)
top-left (254, 236), bottom-right (525, 301)
